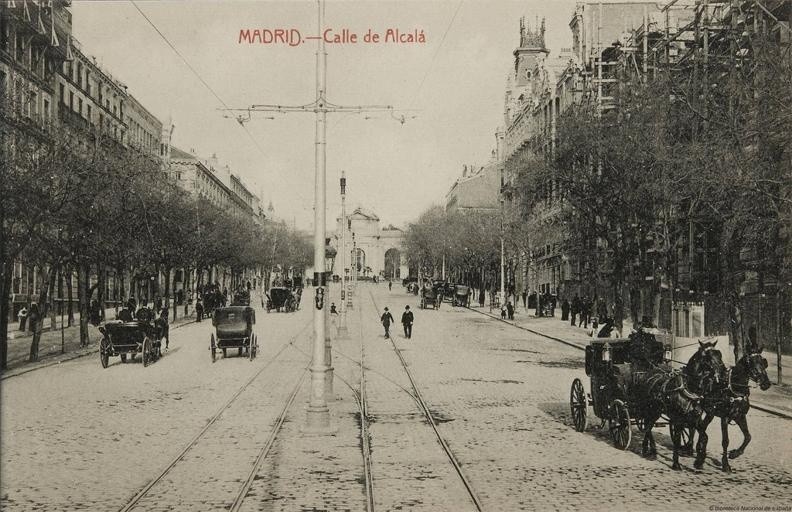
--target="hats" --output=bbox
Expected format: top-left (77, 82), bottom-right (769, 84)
top-left (405, 305), bottom-right (410, 309)
top-left (384, 306), bottom-right (389, 310)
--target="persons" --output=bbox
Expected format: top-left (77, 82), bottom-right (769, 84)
top-left (86, 274), bottom-right (302, 364)
top-left (501, 289), bottom-right (625, 343)
top-left (17, 307), bottom-right (28, 333)
top-left (630, 315), bottom-right (659, 375)
top-left (401, 304), bottom-right (414, 339)
top-left (380, 306), bottom-right (394, 339)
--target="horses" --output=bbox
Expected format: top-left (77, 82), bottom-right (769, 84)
top-left (154, 305), bottom-right (170, 356)
top-left (686, 341), bottom-right (771, 473)
top-left (637, 338), bottom-right (727, 470)
